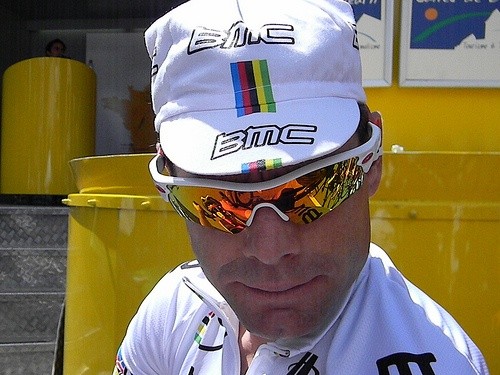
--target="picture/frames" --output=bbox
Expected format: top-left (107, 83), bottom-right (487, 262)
top-left (398, 0), bottom-right (500, 87)
top-left (345, 0), bottom-right (394, 87)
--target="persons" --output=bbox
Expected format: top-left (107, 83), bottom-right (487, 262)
top-left (108, 0), bottom-right (487, 375)
top-left (193, 202), bottom-right (251, 232)
top-left (46, 38), bottom-right (67, 58)
top-left (272, 168), bottom-right (344, 214)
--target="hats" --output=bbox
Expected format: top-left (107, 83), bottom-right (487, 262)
top-left (143, 0), bottom-right (366, 175)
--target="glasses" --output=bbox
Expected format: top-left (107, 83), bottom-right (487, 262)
top-left (147, 122), bottom-right (382, 236)
